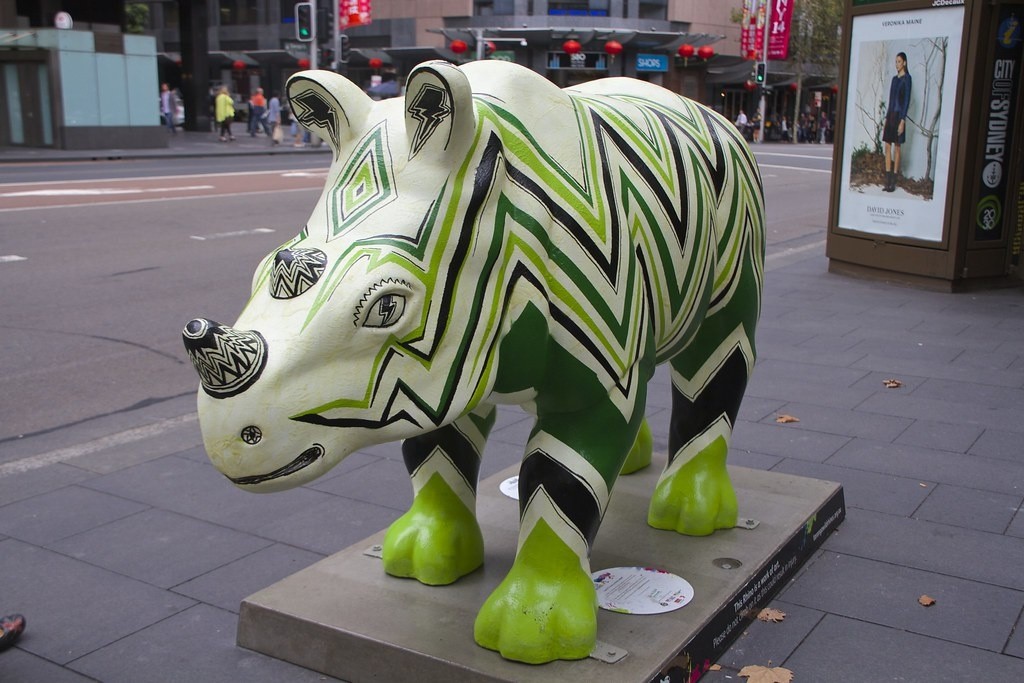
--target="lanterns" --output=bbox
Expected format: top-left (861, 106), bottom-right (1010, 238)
top-left (450, 40), bottom-right (466, 52)
top-left (604, 40), bottom-right (622, 62)
top-left (298, 59), bottom-right (309, 67)
top-left (791, 82), bottom-right (798, 90)
top-left (679, 44), bottom-right (714, 67)
top-left (833, 83), bottom-right (839, 93)
top-left (368, 59), bottom-right (382, 68)
top-left (745, 80), bottom-right (755, 91)
top-left (562, 40), bottom-right (580, 55)
top-left (488, 42), bottom-right (496, 50)
top-left (233, 61), bottom-right (245, 70)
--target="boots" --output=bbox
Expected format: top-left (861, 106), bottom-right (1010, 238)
top-left (882, 171), bottom-right (898, 193)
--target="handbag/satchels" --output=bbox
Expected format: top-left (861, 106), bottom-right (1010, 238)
top-left (273, 125), bottom-right (285, 143)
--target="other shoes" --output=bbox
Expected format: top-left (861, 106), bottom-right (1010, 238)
top-left (218, 137), bottom-right (227, 141)
top-left (229, 136), bottom-right (236, 140)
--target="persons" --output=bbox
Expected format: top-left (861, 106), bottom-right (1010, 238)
top-left (288, 108), bottom-right (323, 147)
top-left (820, 112), bottom-right (830, 144)
top-left (160, 83), bottom-right (178, 134)
top-left (248, 88), bottom-right (271, 137)
top-left (268, 91), bottom-right (283, 147)
top-left (881, 52), bottom-right (912, 192)
top-left (735, 108), bottom-right (747, 133)
top-left (210, 86), bottom-right (236, 141)
top-left (747, 107), bottom-right (762, 142)
top-left (780, 112), bottom-right (817, 144)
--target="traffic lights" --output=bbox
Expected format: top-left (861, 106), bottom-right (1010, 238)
top-left (316, 8), bottom-right (334, 45)
top-left (339, 35), bottom-right (350, 63)
top-left (293, 2), bottom-right (314, 41)
top-left (755, 62), bottom-right (765, 84)
